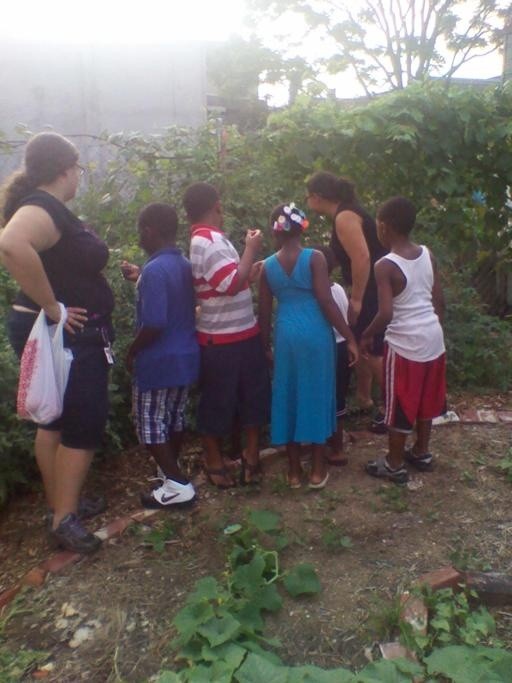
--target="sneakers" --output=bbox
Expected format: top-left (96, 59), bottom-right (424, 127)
top-left (139, 477), bottom-right (196, 508)
top-left (366, 457), bottom-right (409, 484)
top-left (405, 446), bottom-right (436, 472)
top-left (146, 464), bottom-right (171, 485)
top-left (44, 513), bottom-right (103, 552)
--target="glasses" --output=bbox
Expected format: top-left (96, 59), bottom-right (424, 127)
top-left (74, 161), bottom-right (86, 175)
top-left (304, 194), bottom-right (313, 201)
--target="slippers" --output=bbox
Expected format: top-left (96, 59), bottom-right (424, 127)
top-left (325, 448), bottom-right (349, 466)
top-left (206, 462), bottom-right (237, 489)
top-left (306, 470), bottom-right (330, 489)
top-left (285, 466), bottom-right (302, 489)
top-left (241, 451), bottom-right (264, 485)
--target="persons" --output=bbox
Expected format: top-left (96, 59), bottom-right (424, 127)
top-left (121, 205), bottom-right (201, 511)
top-left (257, 203), bottom-right (363, 490)
top-left (302, 169), bottom-right (389, 435)
top-left (317, 250), bottom-right (351, 416)
top-left (183, 183), bottom-right (266, 492)
top-left (363, 196), bottom-right (448, 486)
top-left (1, 132), bottom-right (115, 556)
